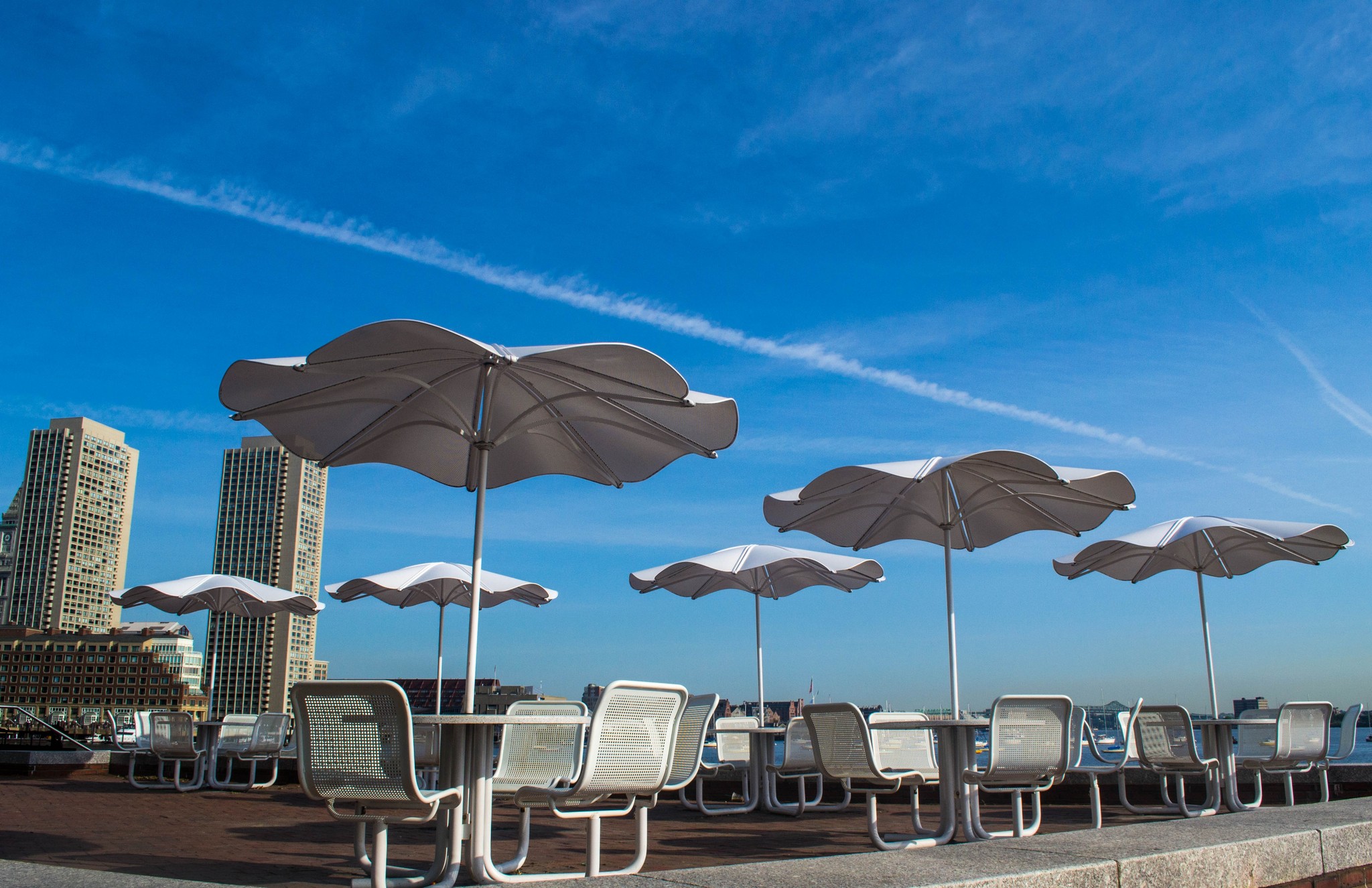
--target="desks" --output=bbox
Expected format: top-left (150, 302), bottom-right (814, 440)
top-left (703, 725), bottom-right (811, 814)
top-left (866, 719), bottom-right (1047, 846)
top-left (1143, 719), bottom-right (1289, 813)
top-left (159, 720), bottom-right (257, 786)
top-left (341, 711), bottom-right (592, 885)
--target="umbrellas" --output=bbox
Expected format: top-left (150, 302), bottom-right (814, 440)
top-left (219, 319), bottom-right (739, 713)
top-left (1052, 516), bottom-right (1355, 718)
top-left (629, 544), bottom-right (886, 727)
top-left (324, 562), bottom-right (559, 715)
top-left (764, 449), bottom-right (1138, 720)
top-left (109, 574), bottom-right (326, 721)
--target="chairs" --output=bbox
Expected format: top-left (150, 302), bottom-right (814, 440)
top-left (290, 678), bottom-right (461, 888)
top-left (104, 706), bottom-right (290, 791)
top-left (492, 680), bottom-right (1364, 881)
